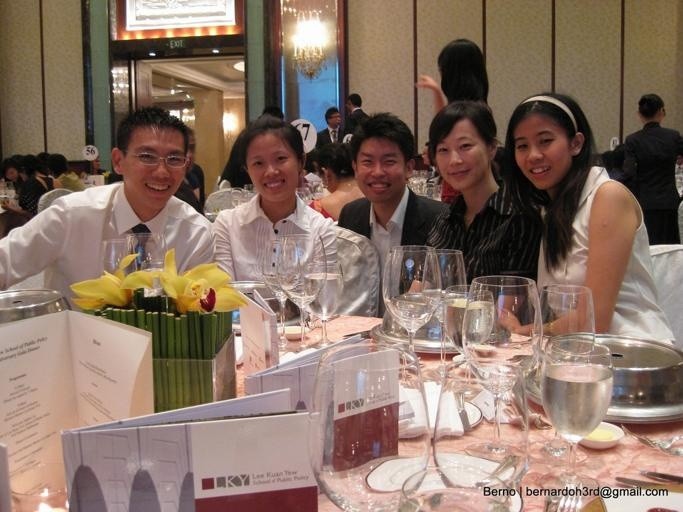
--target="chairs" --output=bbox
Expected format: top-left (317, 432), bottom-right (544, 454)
top-left (204, 186), bottom-right (247, 221)
top-left (35, 187), bottom-right (74, 214)
top-left (331, 223), bottom-right (382, 321)
top-left (646, 240), bottom-right (683, 354)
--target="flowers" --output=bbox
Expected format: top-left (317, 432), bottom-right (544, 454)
top-left (67, 246), bottom-right (246, 317)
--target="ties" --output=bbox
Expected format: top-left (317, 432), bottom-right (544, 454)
top-left (130, 221), bottom-right (152, 274)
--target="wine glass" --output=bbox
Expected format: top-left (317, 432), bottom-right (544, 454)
top-left (0, 232), bottom-right (682, 512)
top-left (674, 162), bottom-right (683, 198)
top-left (227, 169), bottom-right (444, 210)
top-left (0, 178), bottom-right (17, 206)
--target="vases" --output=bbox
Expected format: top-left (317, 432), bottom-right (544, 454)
top-left (94, 308), bottom-right (242, 412)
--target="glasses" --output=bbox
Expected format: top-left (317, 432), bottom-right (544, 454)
top-left (125, 149), bottom-right (191, 165)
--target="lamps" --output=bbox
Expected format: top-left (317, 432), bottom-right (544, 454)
top-left (286, 8), bottom-right (330, 86)
